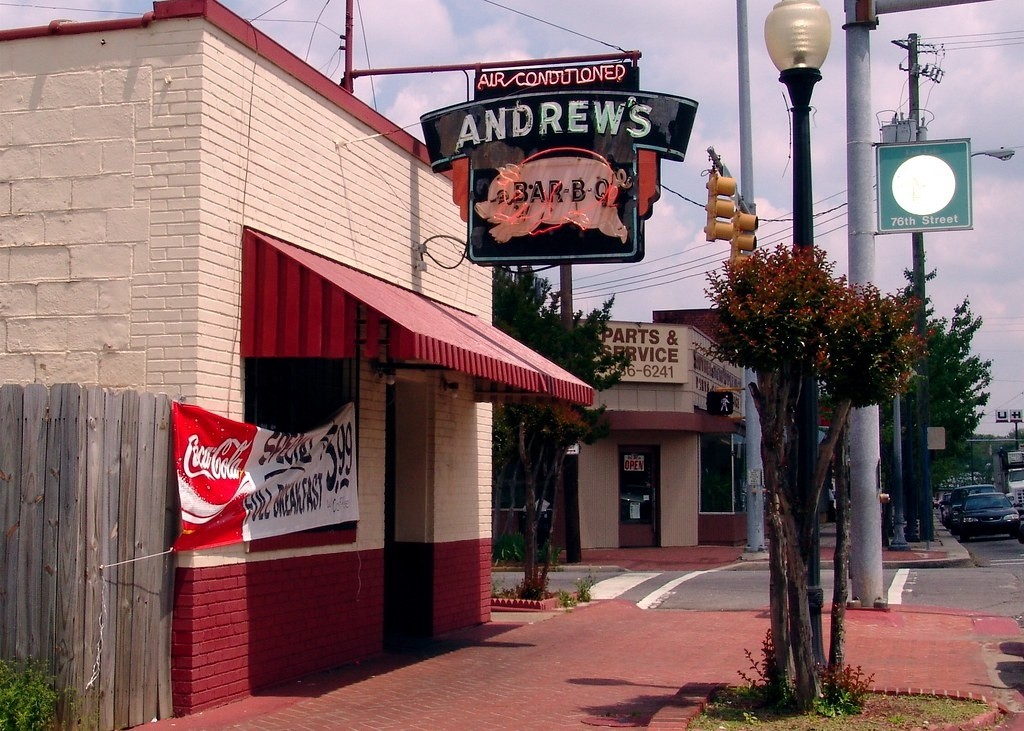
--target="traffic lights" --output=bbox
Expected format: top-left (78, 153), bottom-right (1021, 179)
top-left (704, 172), bottom-right (736, 242)
top-left (729, 209), bottom-right (758, 270)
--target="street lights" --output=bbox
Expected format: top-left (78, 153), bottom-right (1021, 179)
top-left (764, 0), bottom-right (835, 694)
top-left (910, 145), bottom-right (1016, 544)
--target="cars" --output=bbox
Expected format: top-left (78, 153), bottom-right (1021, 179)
top-left (932, 467), bottom-right (1024, 544)
